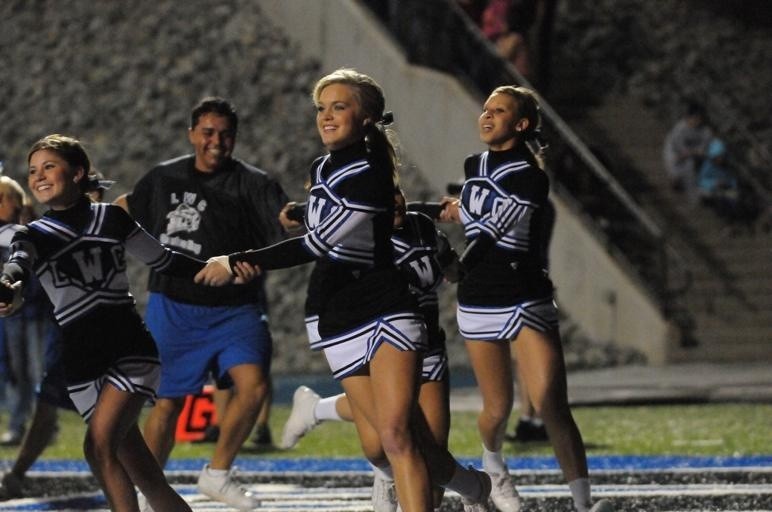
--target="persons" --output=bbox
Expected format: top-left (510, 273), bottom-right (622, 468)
top-left (111, 97), bottom-right (289, 510)
top-left (0, 169), bottom-right (106, 449)
top-left (193, 67), bottom-right (491, 511)
top-left (279, 171), bottom-right (463, 511)
top-left (662, 102), bottom-right (714, 210)
top-left (205, 313), bottom-right (275, 443)
top-left (481, 1), bottom-right (538, 79)
top-left (0, 133), bottom-right (262, 511)
top-left (436, 86), bottom-right (619, 511)
top-left (697, 138), bottom-right (747, 214)
top-left (515, 381), bottom-right (550, 441)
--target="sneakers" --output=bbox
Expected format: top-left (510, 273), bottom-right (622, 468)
top-left (462, 461), bottom-right (493, 512)
top-left (369, 464), bottom-right (403, 512)
top-left (480, 462), bottom-right (523, 511)
top-left (280, 383), bottom-right (323, 452)
top-left (584, 498), bottom-right (614, 512)
top-left (196, 462), bottom-right (264, 511)
